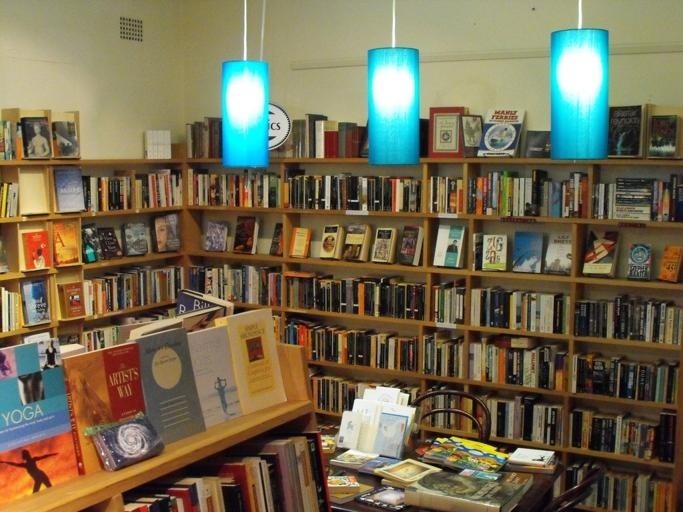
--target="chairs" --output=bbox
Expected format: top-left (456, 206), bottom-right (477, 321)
top-left (543, 468), bottom-right (601, 512)
top-left (410, 389), bottom-right (491, 444)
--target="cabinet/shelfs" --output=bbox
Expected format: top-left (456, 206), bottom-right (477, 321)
top-left (0, 160), bottom-right (185, 351)
top-left (186, 158), bottom-right (683, 510)
top-left (1, 341), bottom-right (330, 512)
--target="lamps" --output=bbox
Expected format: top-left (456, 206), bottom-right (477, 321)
top-left (366, 1), bottom-right (420, 166)
top-left (549, 0), bottom-right (611, 161)
top-left (220, 0), bottom-right (270, 168)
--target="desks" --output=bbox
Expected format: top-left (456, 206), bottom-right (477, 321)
top-left (323, 442), bottom-right (565, 512)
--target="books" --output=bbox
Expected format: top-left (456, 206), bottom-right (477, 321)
top-left (328, 387), bottom-right (439, 510)
top-left (189, 262), bottom-right (283, 308)
top-left (593, 174), bottom-right (683, 224)
top-left (310, 373), bottom-right (420, 423)
top-left (432, 279), bottom-right (465, 326)
top-left (608, 106), bottom-right (680, 160)
top-left (286, 315), bottom-right (420, 374)
top-left (0, 106), bottom-right (81, 161)
top-left (1, 166), bottom-right (51, 220)
top-left (426, 383), bottom-right (459, 427)
top-left (406, 468), bottom-right (533, 511)
top-left (418, 436), bottom-right (559, 473)
top-left (570, 406), bottom-right (676, 464)
top-left (141, 117), bottom-right (223, 159)
top-left (120, 431), bottom-right (330, 511)
top-left (426, 173), bottom-right (465, 217)
top-left (53, 215), bottom-right (183, 269)
top-left (420, 105), bottom-right (551, 160)
top-left (284, 174), bottom-right (423, 213)
top-left (0, 221), bottom-right (50, 275)
top-left (471, 283), bottom-right (572, 335)
top-left (466, 390), bottom-right (563, 447)
top-left (423, 329), bottom-right (464, 379)
top-left (572, 350), bottom-right (680, 406)
top-left (573, 294), bottom-right (683, 348)
top-left (270, 112), bottom-right (369, 160)
top-left (285, 270), bottom-right (426, 321)
top-left (470, 230), bottom-right (573, 277)
top-left (59, 263), bottom-right (185, 320)
top-left (186, 167), bottom-right (282, 212)
top-left (201, 216), bottom-right (283, 258)
top-left (431, 225), bottom-right (466, 271)
top-left (468, 333), bottom-right (567, 388)
top-left (566, 458), bottom-right (674, 511)
top-left (581, 229), bottom-right (682, 284)
top-left (0, 281), bottom-right (289, 508)
top-left (288, 219), bottom-right (425, 266)
top-left (468, 170), bottom-right (588, 220)
top-left (54, 166), bottom-right (184, 215)
